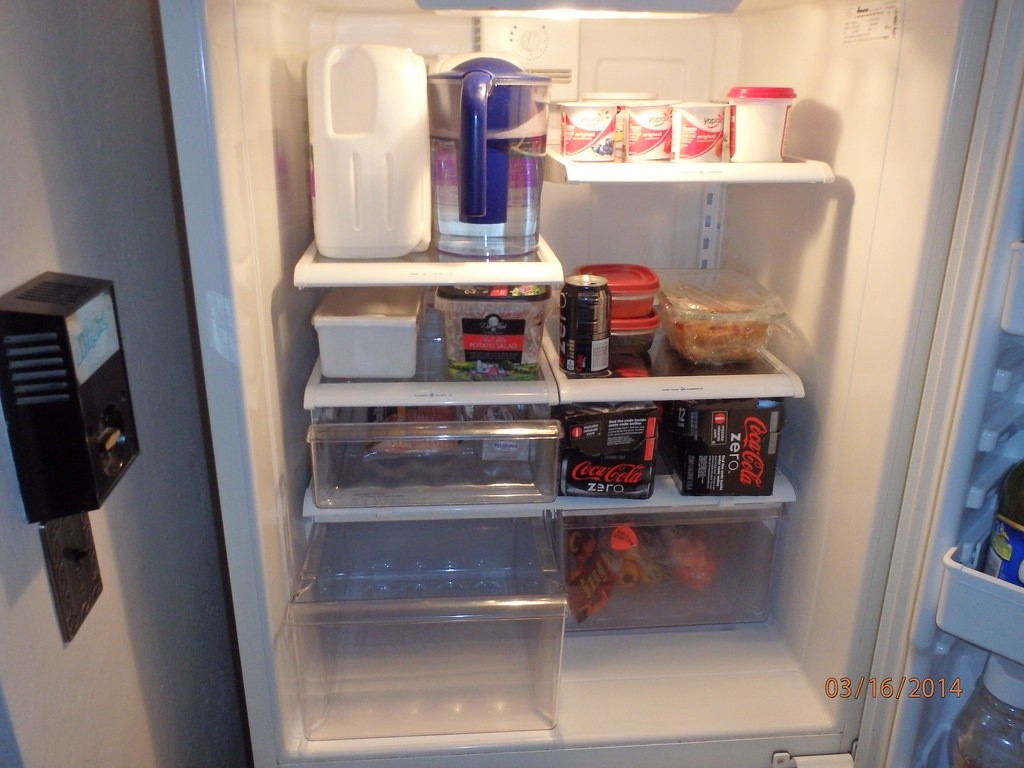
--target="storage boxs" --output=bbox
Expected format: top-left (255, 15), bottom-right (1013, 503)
top-left (309, 287), bottom-right (425, 376)
top-left (434, 286), bottom-right (554, 380)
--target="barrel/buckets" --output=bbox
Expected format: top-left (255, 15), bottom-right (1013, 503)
top-left (306, 47), bottom-right (432, 259)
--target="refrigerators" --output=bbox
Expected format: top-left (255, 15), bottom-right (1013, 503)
top-left (158, 0), bottom-right (1024, 768)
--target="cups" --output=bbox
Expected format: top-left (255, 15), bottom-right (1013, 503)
top-left (727, 88), bottom-right (798, 163)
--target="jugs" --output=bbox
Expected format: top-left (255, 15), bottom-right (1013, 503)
top-left (428, 57), bottom-right (550, 256)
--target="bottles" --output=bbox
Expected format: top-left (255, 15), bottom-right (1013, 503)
top-left (949, 654), bottom-right (1024, 768)
top-left (982, 460), bottom-right (1024, 588)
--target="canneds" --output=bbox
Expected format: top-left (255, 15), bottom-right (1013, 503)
top-left (559, 274), bottom-right (612, 375)
